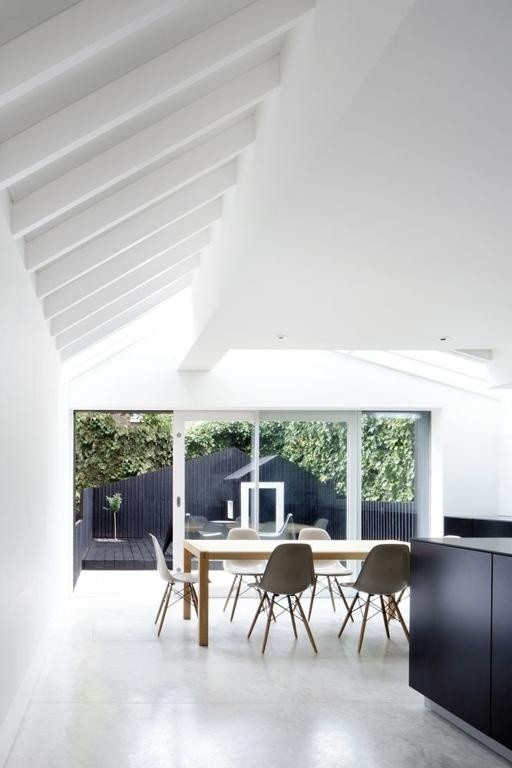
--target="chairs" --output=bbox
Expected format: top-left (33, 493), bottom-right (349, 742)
top-left (184, 512), bottom-right (329, 539)
top-left (148, 533), bottom-right (211, 637)
top-left (222, 528), bottom-right (411, 653)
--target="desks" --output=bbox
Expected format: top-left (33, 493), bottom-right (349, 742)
top-left (184, 539), bottom-right (411, 646)
top-left (409, 537), bottom-right (512, 763)
